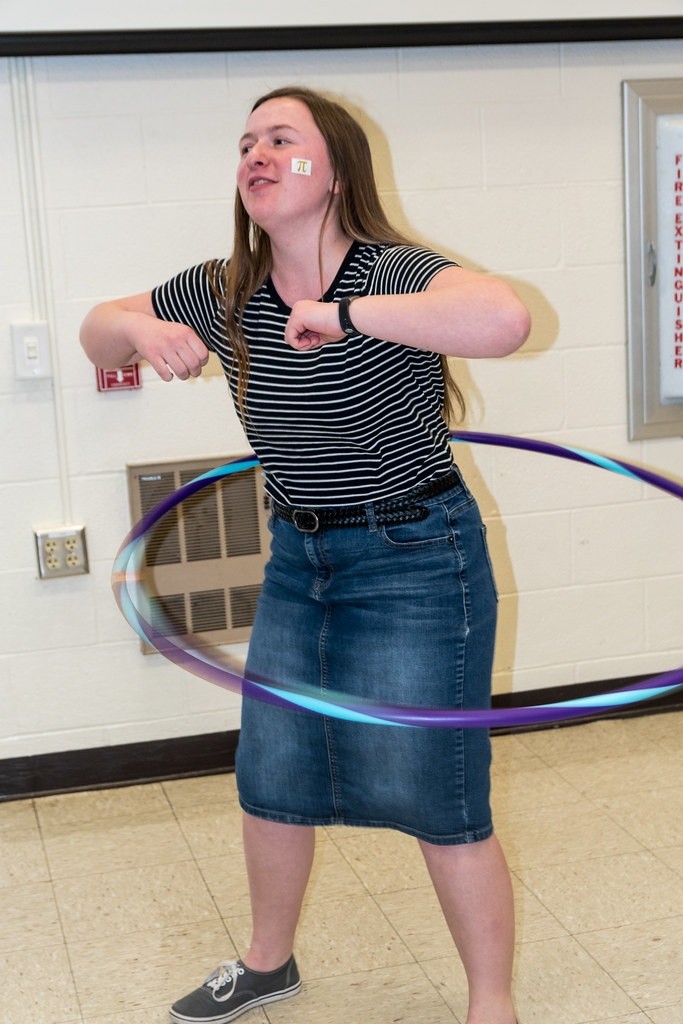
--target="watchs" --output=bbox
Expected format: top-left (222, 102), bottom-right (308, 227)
top-left (339, 293), bottom-right (360, 335)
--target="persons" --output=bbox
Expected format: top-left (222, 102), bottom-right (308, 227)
top-left (79, 87), bottom-right (530, 1024)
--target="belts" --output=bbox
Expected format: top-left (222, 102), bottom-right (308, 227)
top-left (274, 468), bottom-right (461, 533)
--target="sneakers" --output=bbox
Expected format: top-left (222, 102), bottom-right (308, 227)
top-left (167, 953), bottom-right (303, 1024)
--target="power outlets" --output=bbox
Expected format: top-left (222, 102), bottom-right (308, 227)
top-left (35, 526), bottom-right (89, 579)
